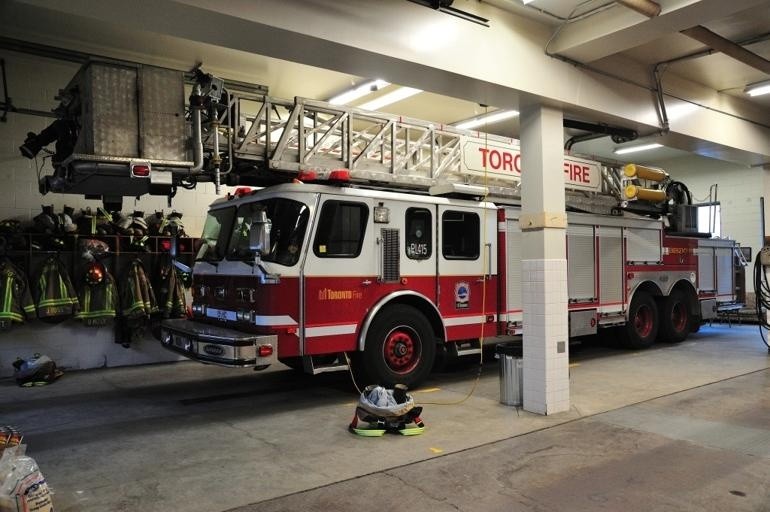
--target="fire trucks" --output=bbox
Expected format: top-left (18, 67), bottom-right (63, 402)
top-left (17, 55), bottom-right (750, 391)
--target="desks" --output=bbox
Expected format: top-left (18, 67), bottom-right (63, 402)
top-left (708, 301), bottom-right (741, 327)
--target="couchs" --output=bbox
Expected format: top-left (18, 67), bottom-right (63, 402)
top-left (713, 290), bottom-right (769, 324)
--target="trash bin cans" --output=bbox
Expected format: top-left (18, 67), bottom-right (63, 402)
top-left (495, 341), bottom-right (523, 407)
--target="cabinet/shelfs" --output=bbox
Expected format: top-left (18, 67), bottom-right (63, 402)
top-left (0, 224), bottom-right (202, 288)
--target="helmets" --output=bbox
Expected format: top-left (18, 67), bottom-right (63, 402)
top-left (83, 262), bottom-right (105, 287)
